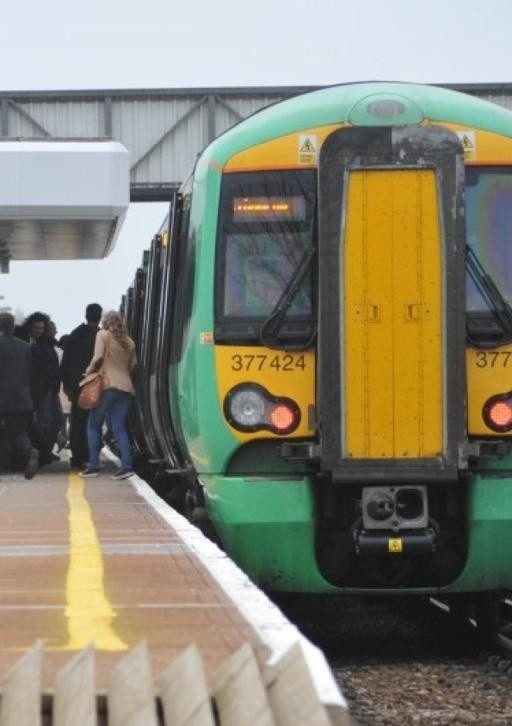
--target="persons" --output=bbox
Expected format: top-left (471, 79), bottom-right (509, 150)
top-left (1, 309), bottom-right (70, 482)
top-left (60, 302), bottom-right (104, 471)
top-left (77, 309), bottom-right (140, 481)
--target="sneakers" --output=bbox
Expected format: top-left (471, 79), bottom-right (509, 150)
top-left (110, 469), bottom-right (133, 481)
top-left (72, 462), bottom-right (99, 478)
top-left (23, 441), bottom-right (67, 479)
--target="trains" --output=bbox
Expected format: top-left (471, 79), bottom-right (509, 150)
top-left (117, 79), bottom-right (511, 638)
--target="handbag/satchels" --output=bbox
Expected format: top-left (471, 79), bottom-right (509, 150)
top-left (76, 373), bottom-right (103, 410)
